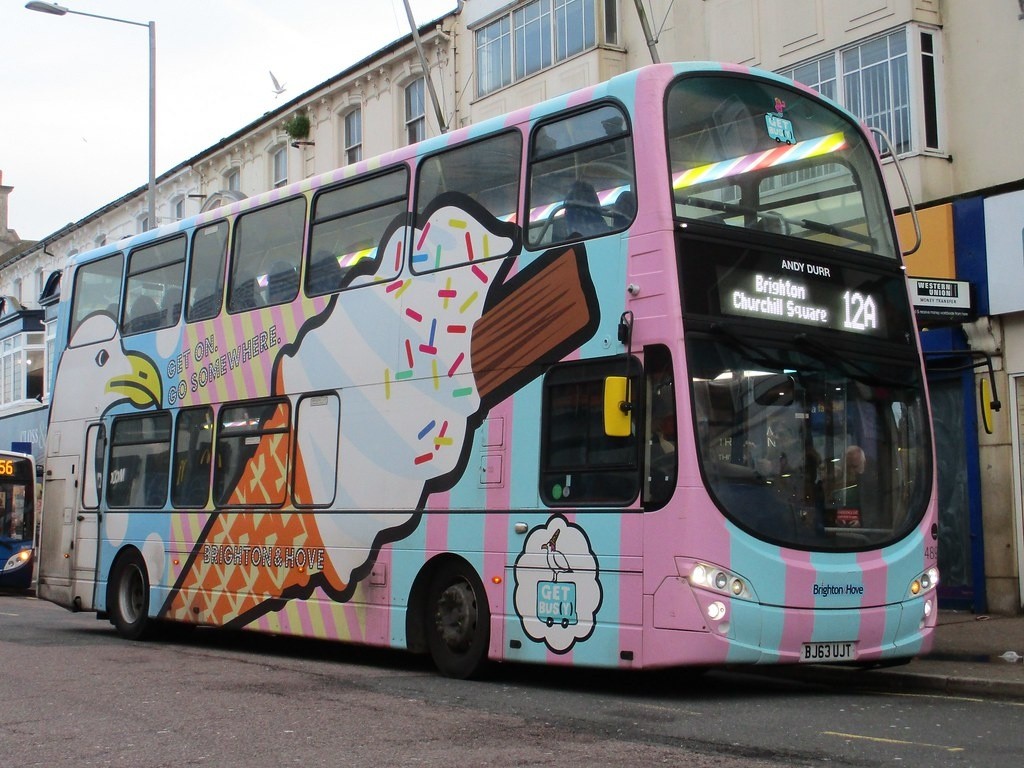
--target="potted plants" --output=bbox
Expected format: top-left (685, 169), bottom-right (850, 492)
top-left (281, 115), bottom-right (310, 139)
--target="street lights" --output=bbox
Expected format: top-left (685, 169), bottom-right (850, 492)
top-left (26, 1), bottom-right (157, 232)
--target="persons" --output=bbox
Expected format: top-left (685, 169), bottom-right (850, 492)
top-left (833, 445), bottom-right (876, 528)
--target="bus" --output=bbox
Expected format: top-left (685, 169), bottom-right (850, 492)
top-left (37, 60), bottom-right (1000, 679)
top-left (0, 449), bottom-right (44, 592)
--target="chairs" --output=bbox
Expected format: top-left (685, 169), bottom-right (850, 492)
top-left (104, 181), bottom-right (633, 337)
